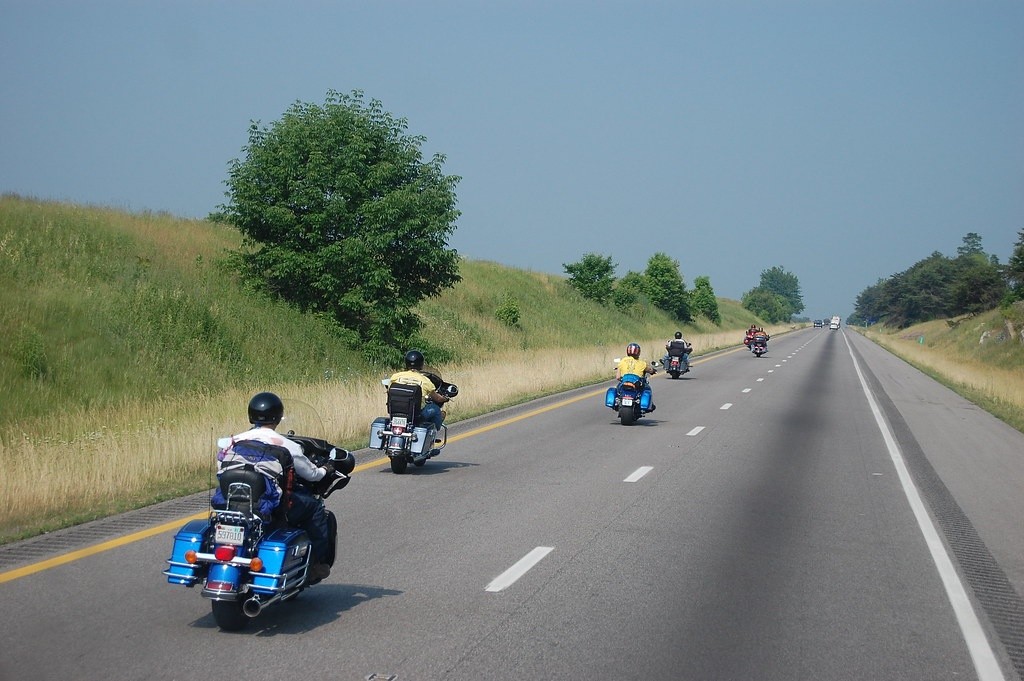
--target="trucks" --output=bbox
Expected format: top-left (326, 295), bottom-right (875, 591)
top-left (831, 317), bottom-right (841, 328)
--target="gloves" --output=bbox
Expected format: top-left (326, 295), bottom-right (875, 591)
top-left (322, 462), bottom-right (335, 477)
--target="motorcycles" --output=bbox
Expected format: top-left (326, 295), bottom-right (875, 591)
top-left (366, 360), bottom-right (458, 473)
top-left (659, 338), bottom-right (693, 378)
top-left (744, 328), bottom-right (770, 358)
top-left (606, 357), bottom-right (659, 425)
top-left (164, 401), bottom-right (355, 631)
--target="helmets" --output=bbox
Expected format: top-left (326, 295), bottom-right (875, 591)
top-left (248, 392), bottom-right (283, 425)
top-left (759, 328), bottom-right (763, 331)
top-left (406, 351), bottom-right (424, 370)
top-left (675, 332), bottom-right (682, 339)
top-left (751, 324), bottom-right (756, 328)
top-left (627, 343), bottom-right (640, 357)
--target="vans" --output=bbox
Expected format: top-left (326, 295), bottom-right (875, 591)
top-left (821, 320), bottom-right (825, 326)
top-left (814, 320), bottom-right (822, 328)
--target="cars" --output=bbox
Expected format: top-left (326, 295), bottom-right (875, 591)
top-left (829, 322), bottom-right (838, 330)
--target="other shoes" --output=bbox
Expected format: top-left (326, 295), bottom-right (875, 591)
top-left (441, 410), bottom-right (446, 422)
top-left (308, 561), bottom-right (329, 582)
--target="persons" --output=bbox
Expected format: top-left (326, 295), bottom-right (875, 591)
top-left (388, 351), bottom-right (447, 431)
top-left (748, 324), bottom-right (768, 353)
top-left (616, 343), bottom-right (656, 412)
top-left (666, 332), bottom-right (690, 364)
top-left (217, 392), bottom-right (336, 584)
